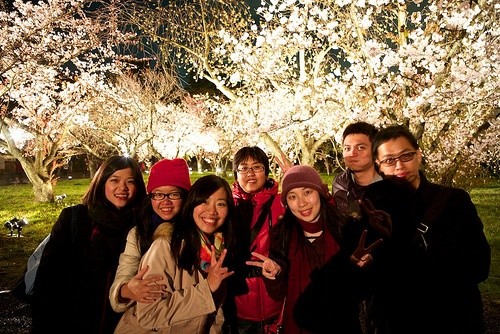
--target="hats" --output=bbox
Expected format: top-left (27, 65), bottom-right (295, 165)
top-left (147, 158), bottom-right (191, 194)
top-left (280, 165), bottom-right (326, 206)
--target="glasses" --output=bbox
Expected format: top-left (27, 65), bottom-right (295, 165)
top-left (380, 151), bottom-right (418, 167)
top-left (150, 191), bottom-right (186, 200)
top-left (237, 166), bottom-right (266, 173)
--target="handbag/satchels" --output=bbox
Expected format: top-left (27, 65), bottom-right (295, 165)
top-left (9, 232), bottom-right (52, 304)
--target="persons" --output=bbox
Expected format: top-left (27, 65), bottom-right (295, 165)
top-left (246, 166), bottom-right (382, 334)
top-left (31, 156), bottom-right (148, 334)
top-left (332, 122), bottom-right (490, 334)
top-left (109, 146), bottom-right (286, 333)
